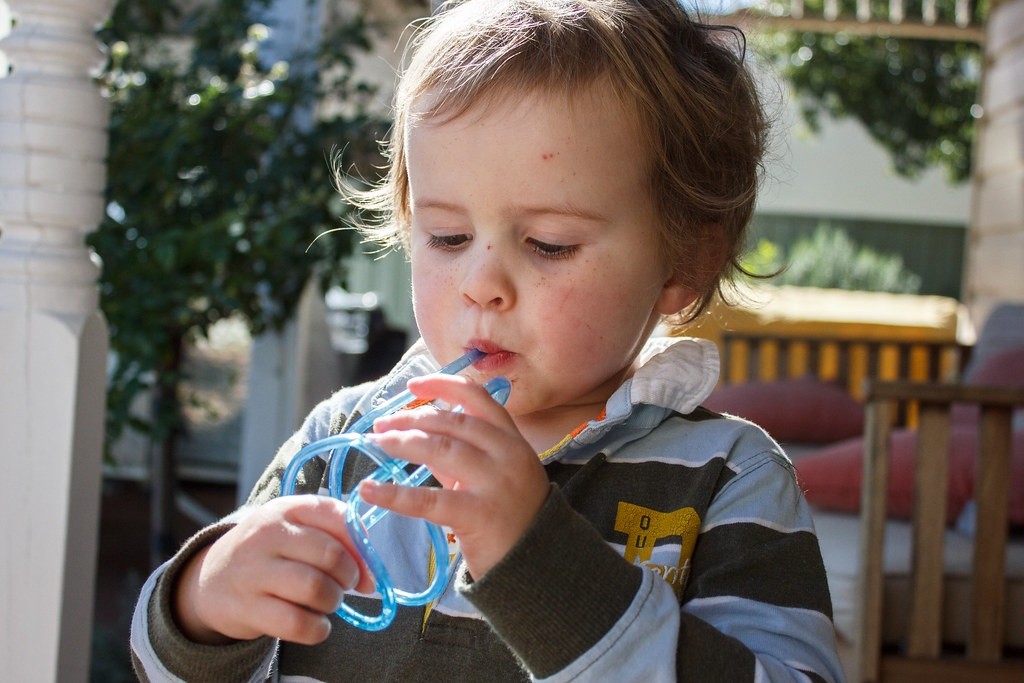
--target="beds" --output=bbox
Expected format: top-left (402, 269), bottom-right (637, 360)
top-left (668, 276), bottom-right (1024, 683)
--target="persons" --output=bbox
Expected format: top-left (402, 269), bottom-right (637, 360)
top-left (129, 0), bottom-right (844, 683)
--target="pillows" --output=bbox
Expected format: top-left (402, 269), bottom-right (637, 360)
top-left (787, 422), bottom-right (1024, 532)
top-left (698, 378), bottom-right (866, 445)
